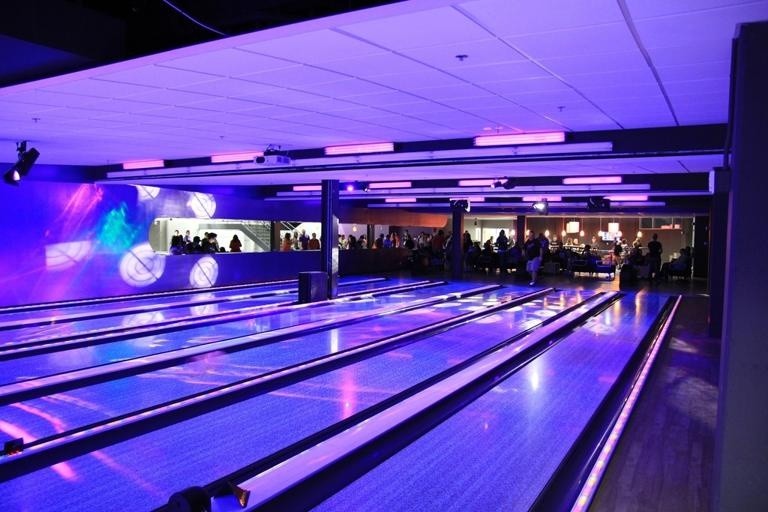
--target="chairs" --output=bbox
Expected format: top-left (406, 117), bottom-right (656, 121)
top-left (574, 258), bottom-right (692, 282)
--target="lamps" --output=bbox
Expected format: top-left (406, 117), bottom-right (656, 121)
top-left (5, 142), bottom-right (40, 186)
top-left (526, 218), bottom-right (643, 238)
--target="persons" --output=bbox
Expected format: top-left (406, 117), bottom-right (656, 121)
top-left (429, 230), bottom-right (445, 278)
top-left (463, 229), bottom-right (521, 261)
top-left (172, 230), bottom-right (242, 253)
top-left (521, 231), bottom-right (543, 285)
top-left (583, 231), bottom-right (694, 290)
top-left (536, 233), bottom-right (568, 267)
top-left (283, 230), bottom-right (320, 251)
top-left (338, 230), bottom-right (432, 250)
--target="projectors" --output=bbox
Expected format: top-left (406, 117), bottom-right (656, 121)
top-left (252, 154), bottom-right (291, 167)
